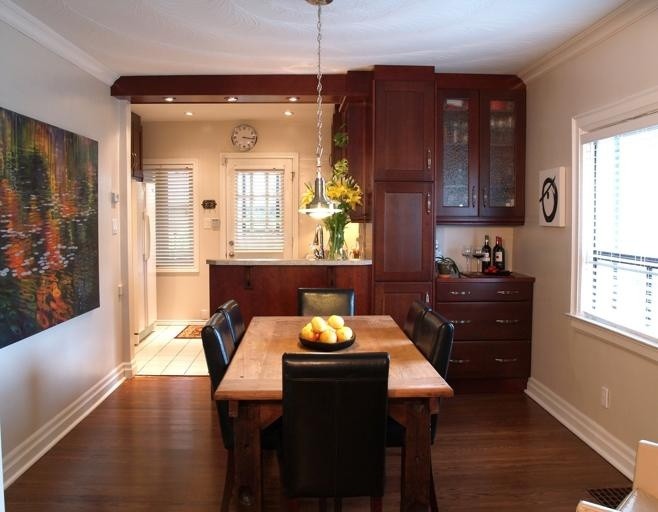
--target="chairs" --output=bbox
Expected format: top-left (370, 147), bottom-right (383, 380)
top-left (271, 352), bottom-right (389, 511)
top-left (199, 311), bottom-right (281, 511)
top-left (400, 299), bottom-right (432, 346)
top-left (574, 438), bottom-right (658, 512)
top-left (214, 299), bottom-right (245, 350)
top-left (381, 308), bottom-right (455, 512)
top-left (298, 287), bottom-right (355, 315)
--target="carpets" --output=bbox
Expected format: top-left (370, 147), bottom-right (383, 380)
top-left (584, 485), bottom-right (633, 510)
top-left (175, 324), bottom-right (204, 340)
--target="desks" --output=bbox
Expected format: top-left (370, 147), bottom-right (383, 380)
top-left (205, 258), bottom-right (372, 333)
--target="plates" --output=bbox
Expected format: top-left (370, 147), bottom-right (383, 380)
top-left (298, 329), bottom-right (355, 350)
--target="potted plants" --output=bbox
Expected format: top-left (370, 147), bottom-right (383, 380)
top-left (433, 251), bottom-right (461, 280)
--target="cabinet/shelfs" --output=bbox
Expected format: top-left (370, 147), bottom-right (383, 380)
top-left (371, 285), bottom-right (432, 333)
top-left (372, 180), bottom-right (434, 284)
top-left (434, 276), bottom-right (536, 394)
top-left (371, 63), bottom-right (436, 184)
top-left (435, 72), bottom-right (526, 226)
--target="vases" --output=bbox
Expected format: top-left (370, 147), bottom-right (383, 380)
top-left (324, 227), bottom-right (350, 261)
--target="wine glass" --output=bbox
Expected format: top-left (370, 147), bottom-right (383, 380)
top-left (473, 246), bottom-right (484, 276)
top-left (461, 246), bottom-right (473, 275)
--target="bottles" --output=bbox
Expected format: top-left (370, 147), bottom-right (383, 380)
top-left (481, 234), bottom-right (491, 272)
top-left (493, 236), bottom-right (499, 266)
top-left (494, 237), bottom-right (505, 269)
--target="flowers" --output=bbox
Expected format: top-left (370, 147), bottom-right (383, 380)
top-left (300, 160), bottom-right (365, 233)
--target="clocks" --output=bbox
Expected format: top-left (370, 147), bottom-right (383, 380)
top-left (230, 123), bottom-right (258, 152)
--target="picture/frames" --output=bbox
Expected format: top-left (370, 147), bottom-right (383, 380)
top-left (0, 107), bottom-right (100, 350)
top-left (129, 111), bottom-right (143, 183)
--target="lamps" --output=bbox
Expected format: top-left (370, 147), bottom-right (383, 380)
top-left (297, 1), bottom-right (342, 221)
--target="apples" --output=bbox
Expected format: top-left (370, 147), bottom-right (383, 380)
top-left (302, 314), bottom-right (353, 344)
